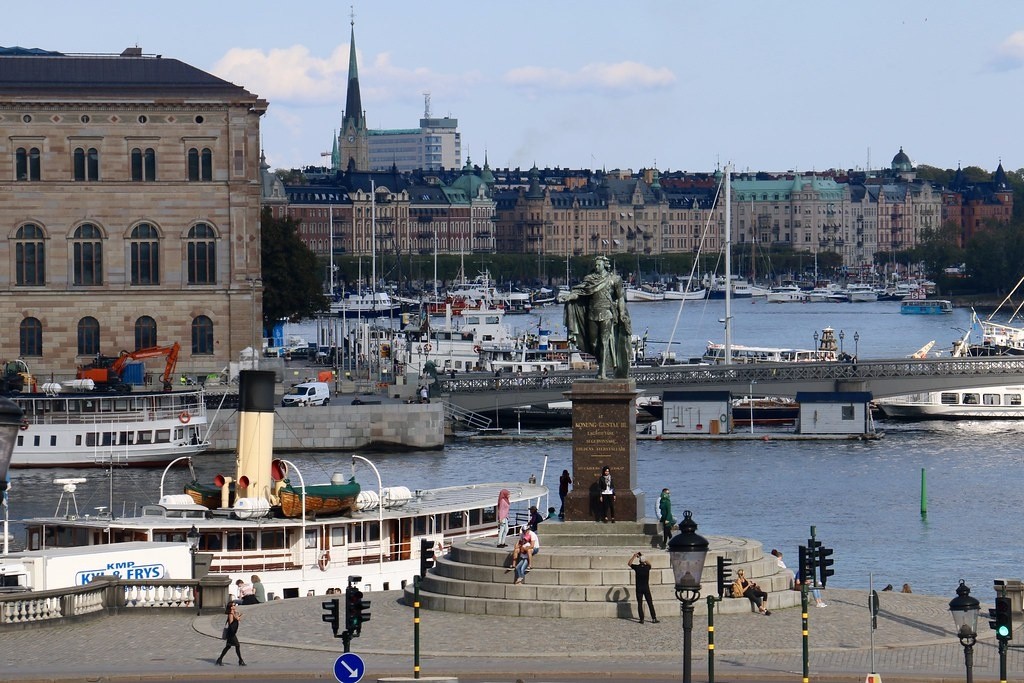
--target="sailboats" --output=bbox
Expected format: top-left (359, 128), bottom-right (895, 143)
top-left (326, 158), bottom-right (1024, 423)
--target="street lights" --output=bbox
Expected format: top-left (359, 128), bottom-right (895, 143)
top-left (839, 330), bottom-right (844, 352)
top-left (854, 331), bottom-right (860, 363)
top-left (947, 578), bottom-right (982, 683)
top-left (665, 510), bottom-right (710, 683)
top-left (813, 331), bottom-right (820, 362)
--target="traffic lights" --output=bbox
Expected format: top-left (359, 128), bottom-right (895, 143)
top-left (818, 545), bottom-right (835, 588)
top-left (322, 598), bottom-right (340, 630)
top-left (988, 608), bottom-right (998, 630)
top-left (995, 597), bottom-right (1013, 641)
top-left (345, 590), bottom-right (363, 631)
top-left (716, 556), bottom-right (734, 595)
top-left (798, 545), bottom-right (815, 582)
top-left (807, 539), bottom-right (822, 568)
top-left (359, 600), bottom-right (371, 623)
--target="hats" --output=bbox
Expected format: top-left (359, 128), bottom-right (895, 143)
top-left (521, 526), bottom-right (530, 530)
top-left (523, 535), bottom-right (531, 541)
top-left (528, 506), bottom-right (537, 510)
top-left (637, 552), bottom-right (644, 558)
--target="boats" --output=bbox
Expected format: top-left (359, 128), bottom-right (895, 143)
top-left (6, 377), bottom-right (213, 468)
top-left (2, 453), bottom-right (552, 605)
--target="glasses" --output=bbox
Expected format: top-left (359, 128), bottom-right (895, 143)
top-left (737, 573), bottom-right (743, 576)
top-left (233, 603), bottom-right (236, 606)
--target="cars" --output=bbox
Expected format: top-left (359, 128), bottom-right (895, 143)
top-left (315, 352), bottom-right (327, 359)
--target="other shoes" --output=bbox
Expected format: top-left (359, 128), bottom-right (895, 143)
top-left (822, 603), bottom-right (827, 606)
top-left (652, 618), bottom-right (659, 623)
top-left (497, 544), bottom-right (504, 548)
top-left (816, 603), bottom-right (825, 608)
top-left (502, 543), bottom-right (508, 546)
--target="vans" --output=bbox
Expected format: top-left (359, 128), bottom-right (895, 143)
top-left (281, 381), bottom-right (330, 408)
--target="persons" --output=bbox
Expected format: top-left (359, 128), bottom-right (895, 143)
top-left (599, 466), bottom-right (617, 522)
top-left (214, 600), bottom-right (246, 667)
top-left (508, 505), bottom-right (557, 584)
top-left (771, 549), bottom-right (787, 569)
top-left (882, 583), bottom-right (912, 594)
top-left (659, 488), bottom-right (677, 549)
top-left (233, 575), bottom-right (266, 606)
top-left (180, 372), bottom-right (187, 384)
top-left (794, 564), bottom-right (827, 608)
top-left (731, 569), bottom-right (772, 616)
top-left (557, 255), bottom-right (628, 378)
top-left (497, 489), bottom-right (511, 548)
top-left (408, 366), bottom-right (550, 404)
top-left (628, 551), bottom-right (661, 624)
top-left (558, 470), bottom-right (572, 517)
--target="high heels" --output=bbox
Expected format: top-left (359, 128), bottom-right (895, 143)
top-left (239, 661), bottom-right (247, 666)
top-left (216, 658), bottom-right (224, 666)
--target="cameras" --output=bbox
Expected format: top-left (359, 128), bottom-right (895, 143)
top-left (637, 552), bottom-right (642, 556)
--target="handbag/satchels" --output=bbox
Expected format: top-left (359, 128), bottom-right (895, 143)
top-left (796, 584), bottom-right (802, 591)
top-left (222, 628), bottom-right (228, 640)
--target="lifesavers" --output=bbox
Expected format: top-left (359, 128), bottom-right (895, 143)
top-left (179, 412), bottom-right (191, 424)
top-left (20, 418), bottom-right (29, 431)
top-left (424, 343), bottom-right (433, 352)
top-left (318, 550), bottom-right (332, 572)
top-left (720, 414), bottom-right (727, 422)
top-left (473, 344), bottom-right (481, 354)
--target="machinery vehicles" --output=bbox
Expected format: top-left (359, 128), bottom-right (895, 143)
top-left (76, 341), bottom-right (182, 394)
